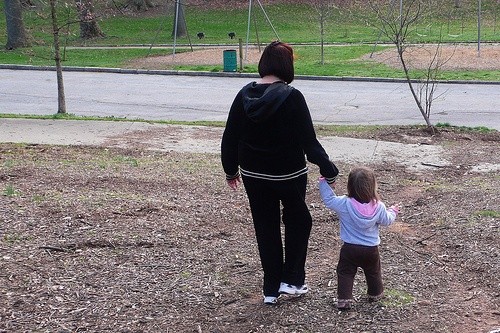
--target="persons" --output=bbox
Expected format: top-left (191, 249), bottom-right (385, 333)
top-left (319, 166), bottom-right (399, 311)
top-left (221, 40), bottom-right (340, 305)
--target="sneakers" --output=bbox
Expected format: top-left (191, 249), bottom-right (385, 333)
top-left (261, 290), bottom-right (281, 303)
top-left (278, 282), bottom-right (308, 295)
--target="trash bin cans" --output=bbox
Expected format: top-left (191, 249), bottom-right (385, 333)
top-left (223, 50), bottom-right (237, 72)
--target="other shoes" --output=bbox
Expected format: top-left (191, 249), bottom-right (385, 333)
top-left (369, 293), bottom-right (384, 301)
top-left (338, 299), bottom-right (353, 309)
